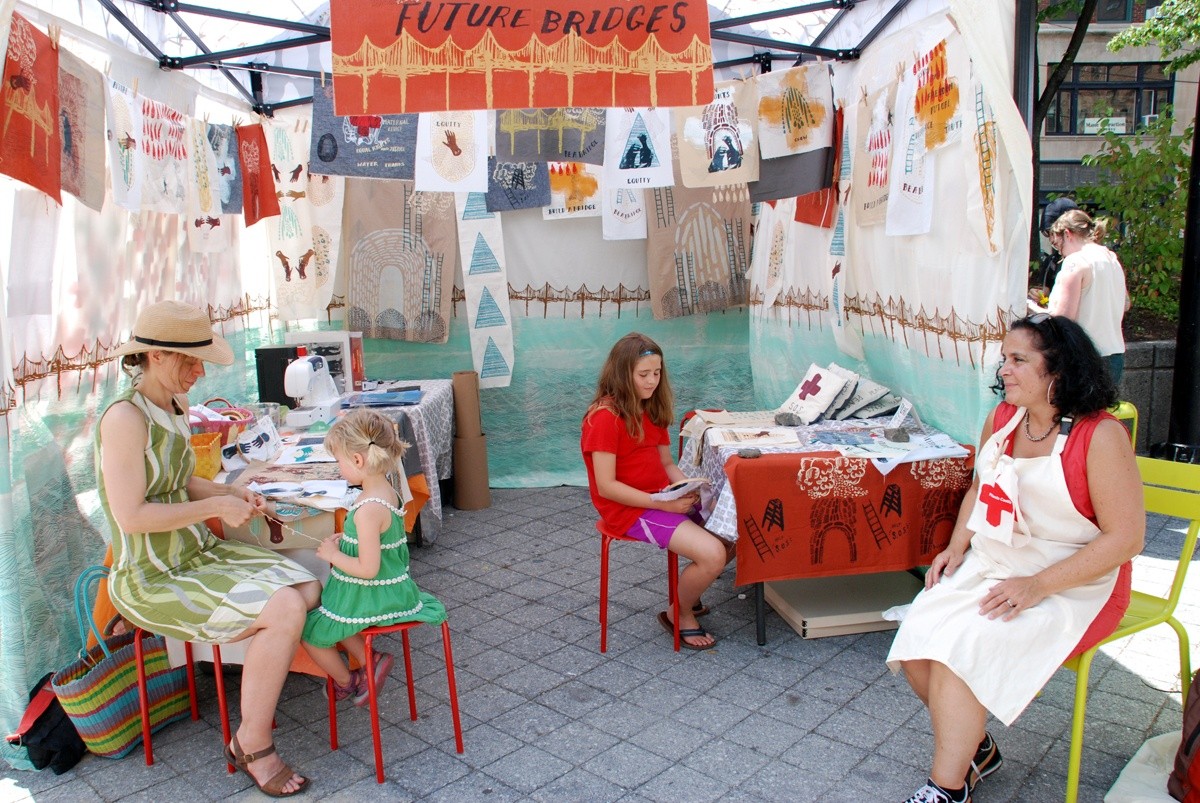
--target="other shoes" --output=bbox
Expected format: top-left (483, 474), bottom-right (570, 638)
top-left (323, 673), bottom-right (364, 703)
top-left (348, 652), bottom-right (394, 707)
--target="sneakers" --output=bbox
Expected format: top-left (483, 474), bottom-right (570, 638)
top-left (966, 731), bottom-right (1003, 792)
top-left (901, 778), bottom-right (972, 803)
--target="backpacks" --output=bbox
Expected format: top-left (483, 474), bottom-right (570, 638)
top-left (1165, 668), bottom-right (1200, 803)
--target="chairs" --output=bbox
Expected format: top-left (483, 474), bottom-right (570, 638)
top-left (1037, 456), bottom-right (1200, 803)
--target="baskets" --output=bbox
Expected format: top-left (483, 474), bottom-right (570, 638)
top-left (189, 397), bottom-right (253, 446)
top-left (190, 432), bottom-right (223, 480)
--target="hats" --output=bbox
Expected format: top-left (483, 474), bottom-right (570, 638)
top-left (105, 301), bottom-right (234, 366)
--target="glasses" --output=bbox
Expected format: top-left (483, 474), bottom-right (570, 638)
top-left (1029, 312), bottom-right (1062, 343)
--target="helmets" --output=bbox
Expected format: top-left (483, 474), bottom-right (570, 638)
top-left (1039, 198), bottom-right (1077, 229)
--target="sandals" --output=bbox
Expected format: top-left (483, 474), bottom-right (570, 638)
top-left (223, 734), bottom-right (310, 797)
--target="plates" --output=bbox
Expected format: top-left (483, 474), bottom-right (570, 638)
top-left (256, 483), bottom-right (304, 497)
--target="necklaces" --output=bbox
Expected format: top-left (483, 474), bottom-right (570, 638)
top-left (1024, 412), bottom-right (1060, 442)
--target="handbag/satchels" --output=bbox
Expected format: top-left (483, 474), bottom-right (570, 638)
top-left (7, 672), bottom-right (90, 775)
top-left (52, 566), bottom-right (193, 759)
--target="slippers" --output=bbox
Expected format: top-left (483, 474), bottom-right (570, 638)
top-left (693, 602), bottom-right (709, 615)
top-left (658, 611), bottom-right (716, 650)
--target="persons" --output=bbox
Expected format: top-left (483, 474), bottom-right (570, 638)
top-left (582, 331), bottom-right (736, 650)
top-left (1028, 197), bottom-right (1131, 388)
top-left (300, 409), bottom-right (448, 708)
top-left (886, 313), bottom-right (1146, 803)
top-left (94, 300), bottom-right (323, 797)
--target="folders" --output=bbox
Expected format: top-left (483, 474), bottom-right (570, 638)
top-left (340, 389), bottom-right (422, 409)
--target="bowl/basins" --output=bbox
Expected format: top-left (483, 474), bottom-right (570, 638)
top-left (247, 403), bottom-right (279, 435)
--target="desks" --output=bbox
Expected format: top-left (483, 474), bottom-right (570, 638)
top-left (678, 413), bottom-right (976, 636)
top-left (163, 377), bottom-right (455, 678)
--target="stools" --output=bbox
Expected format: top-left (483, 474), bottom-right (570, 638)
top-left (327, 620), bottom-right (462, 785)
top-left (679, 409), bottom-right (728, 459)
top-left (596, 521), bottom-right (682, 654)
top-left (133, 628), bottom-right (279, 773)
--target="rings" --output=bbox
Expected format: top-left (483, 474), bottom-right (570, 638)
top-left (1006, 599), bottom-right (1014, 608)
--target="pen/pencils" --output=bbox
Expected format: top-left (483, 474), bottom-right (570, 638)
top-left (662, 482), bottom-right (688, 493)
top-left (391, 379), bottom-right (399, 384)
